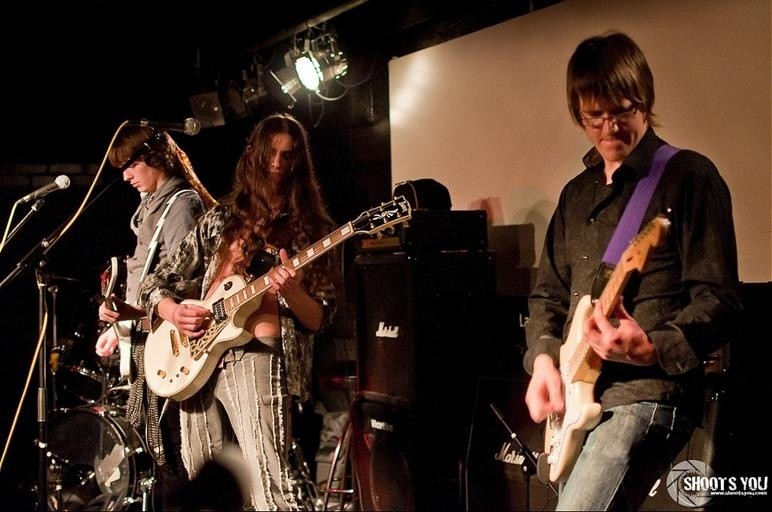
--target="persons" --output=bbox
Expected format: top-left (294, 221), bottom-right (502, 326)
top-left (523, 31), bottom-right (739, 511)
top-left (95, 121), bottom-right (206, 359)
top-left (136, 114), bottom-right (343, 512)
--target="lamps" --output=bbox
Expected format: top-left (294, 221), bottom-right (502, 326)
top-left (188, 15), bottom-right (351, 134)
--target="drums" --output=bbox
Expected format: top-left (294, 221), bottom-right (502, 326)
top-left (32, 402), bottom-right (150, 512)
top-left (58, 361), bottom-right (105, 399)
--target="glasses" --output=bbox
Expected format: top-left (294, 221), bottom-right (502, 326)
top-left (579, 104), bottom-right (641, 128)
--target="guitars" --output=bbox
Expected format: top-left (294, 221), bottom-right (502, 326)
top-left (544, 213), bottom-right (672, 483)
top-left (143, 196), bottom-right (414, 404)
top-left (100, 257), bottom-right (133, 378)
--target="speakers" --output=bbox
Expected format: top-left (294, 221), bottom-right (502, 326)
top-left (355, 251), bottom-right (496, 407)
top-left (352, 403), bottom-right (495, 512)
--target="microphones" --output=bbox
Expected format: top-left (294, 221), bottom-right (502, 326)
top-left (18, 174), bottom-right (70, 204)
top-left (128, 117), bottom-right (201, 137)
